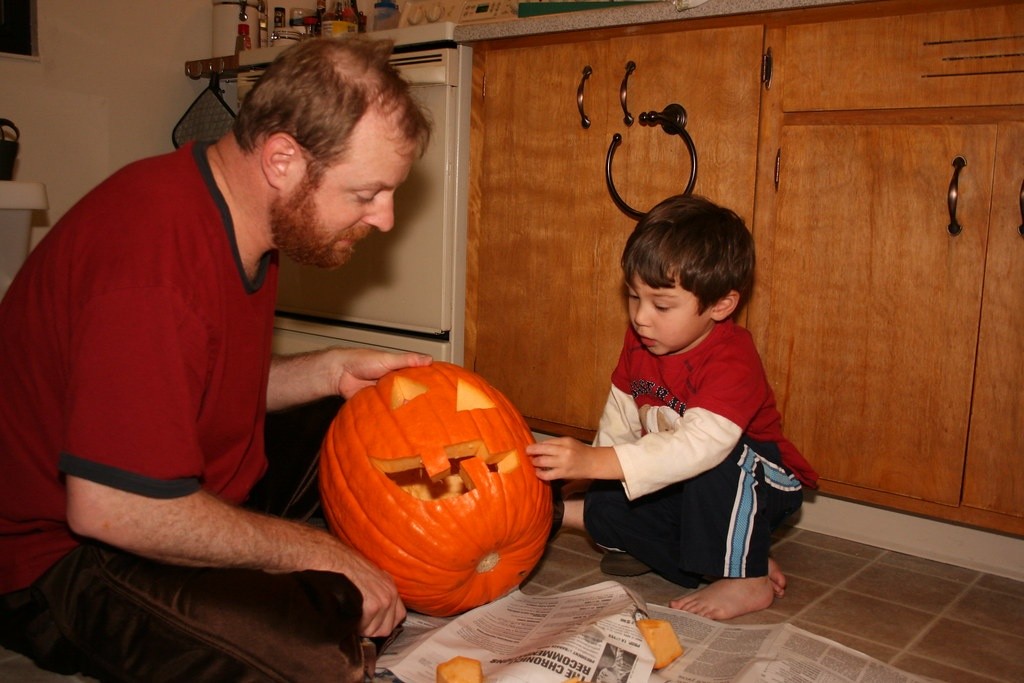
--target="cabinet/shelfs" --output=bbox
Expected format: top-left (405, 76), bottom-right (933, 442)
top-left (465, 0), bottom-right (1024, 543)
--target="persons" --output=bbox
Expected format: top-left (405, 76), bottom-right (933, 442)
top-left (525, 195), bottom-right (820, 620)
top-left (596, 644), bottom-right (637, 683)
top-left (0, 37), bottom-right (433, 683)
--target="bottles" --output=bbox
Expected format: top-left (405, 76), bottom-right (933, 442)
top-left (235, 23), bottom-right (251, 55)
top-left (322, 2), bottom-right (368, 35)
top-left (315, 7), bottom-right (323, 37)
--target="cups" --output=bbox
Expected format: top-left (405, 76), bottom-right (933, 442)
top-left (289, 8), bottom-right (304, 26)
top-left (373, 2), bottom-right (398, 31)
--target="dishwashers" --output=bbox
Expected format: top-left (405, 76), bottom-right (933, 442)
top-left (234, 48), bottom-right (472, 363)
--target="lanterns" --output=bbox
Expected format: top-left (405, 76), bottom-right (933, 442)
top-left (320, 361), bottom-right (554, 617)
